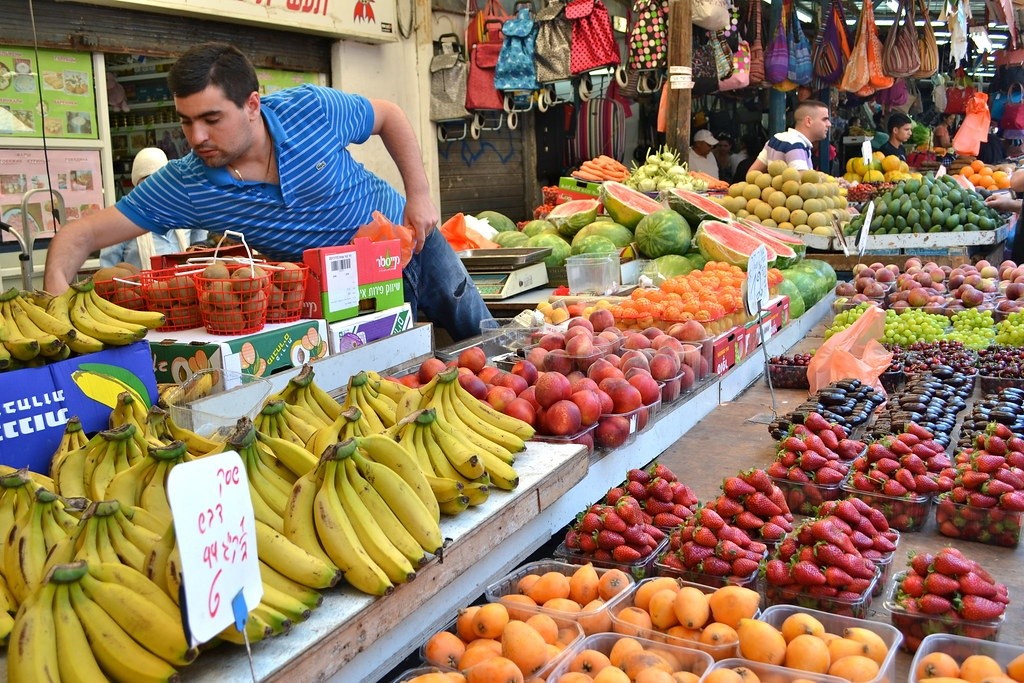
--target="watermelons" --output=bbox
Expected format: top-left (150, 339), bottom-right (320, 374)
top-left (471, 182), bottom-right (838, 318)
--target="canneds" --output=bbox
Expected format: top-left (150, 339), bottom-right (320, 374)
top-left (110, 111), bottom-right (174, 128)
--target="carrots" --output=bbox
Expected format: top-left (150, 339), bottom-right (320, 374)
top-left (571, 154), bottom-right (631, 182)
top-left (689, 171), bottom-right (730, 190)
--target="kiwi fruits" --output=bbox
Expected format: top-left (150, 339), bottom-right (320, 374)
top-left (90, 263), bottom-right (304, 334)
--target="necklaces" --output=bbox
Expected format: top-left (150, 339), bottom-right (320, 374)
top-left (228, 133), bottom-right (273, 182)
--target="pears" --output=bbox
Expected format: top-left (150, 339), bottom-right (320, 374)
top-left (706, 158), bottom-right (850, 236)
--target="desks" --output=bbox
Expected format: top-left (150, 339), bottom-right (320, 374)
top-left (807, 239), bottom-right (1007, 273)
top-left (328, 281), bottom-right (845, 683)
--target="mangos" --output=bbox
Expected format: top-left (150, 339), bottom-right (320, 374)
top-left (841, 172), bottom-right (1003, 236)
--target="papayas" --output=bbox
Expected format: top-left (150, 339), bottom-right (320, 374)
top-left (842, 151), bottom-right (925, 183)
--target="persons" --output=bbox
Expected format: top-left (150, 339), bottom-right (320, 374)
top-left (985, 163), bottom-right (1024, 267)
top-left (838, 116), bottom-right (861, 150)
top-left (99, 147), bottom-right (209, 272)
top-left (44, 42), bottom-right (499, 343)
top-left (689, 130), bottom-right (720, 180)
top-left (876, 113), bottom-right (913, 163)
top-left (748, 100), bottom-right (832, 174)
top-left (713, 133), bottom-right (762, 184)
top-left (933, 112), bottom-right (955, 147)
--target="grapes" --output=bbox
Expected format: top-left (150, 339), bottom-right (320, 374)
top-left (808, 301), bottom-right (1024, 351)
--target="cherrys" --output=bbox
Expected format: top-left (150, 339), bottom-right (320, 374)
top-left (764, 338), bottom-right (1024, 396)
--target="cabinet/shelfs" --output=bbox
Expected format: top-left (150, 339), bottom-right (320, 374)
top-left (102, 59), bottom-right (189, 192)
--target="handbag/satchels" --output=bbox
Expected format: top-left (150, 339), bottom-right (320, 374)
top-left (807, 306), bottom-right (893, 401)
top-left (691, 0), bottom-right (1024, 129)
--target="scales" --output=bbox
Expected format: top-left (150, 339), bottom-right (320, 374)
top-left (456, 247), bottom-right (554, 299)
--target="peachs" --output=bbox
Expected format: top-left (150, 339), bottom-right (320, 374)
top-left (387, 309), bottom-right (711, 452)
top-left (834, 258), bottom-right (1024, 323)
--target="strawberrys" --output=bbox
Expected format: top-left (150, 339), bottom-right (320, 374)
top-left (565, 457), bottom-right (1010, 660)
top-left (767, 411), bottom-right (1024, 548)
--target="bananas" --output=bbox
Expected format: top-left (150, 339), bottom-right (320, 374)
top-left (0, 276), bottom-right (167, 372)
top-left (0, 364), bottom-right (536, 683)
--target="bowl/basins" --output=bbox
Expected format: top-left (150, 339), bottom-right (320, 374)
top-left (564, 253), bottom-right (622, 298)
top-left (762, 285), bottom-right (1024, 384)
top-left (478, 318), bottom-right (714, 458)
top-left (387, 528), bottom-right (1024, 682)
top-left (759, 435), bottom-right (871, 518)
top-left (167, 367), bottom-right (273, 448)
top-left (934, 495), bottom-right (1023, 546)
top-left (840, 471), bottom-right (931, 534)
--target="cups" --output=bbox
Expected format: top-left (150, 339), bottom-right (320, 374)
top-left (637, 260), bottom-right (658, 289)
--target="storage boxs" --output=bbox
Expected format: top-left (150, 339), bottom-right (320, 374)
top-left (0, 210), bottom-right (1024, 683)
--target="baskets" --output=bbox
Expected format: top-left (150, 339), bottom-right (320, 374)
top-left (95, 230), bottom-right (309, 335)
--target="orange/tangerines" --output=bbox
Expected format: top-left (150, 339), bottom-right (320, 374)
top-left (952, 159), bottom-right (1011, 191)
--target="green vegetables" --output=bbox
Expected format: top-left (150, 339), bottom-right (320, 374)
top-left (909, 125), bottom-right (933, 146)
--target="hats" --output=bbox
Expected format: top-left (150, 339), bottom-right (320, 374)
top-left (694, 130), bottom-right (719, 146)
top-left (132, 147), bottom-right (167, 186)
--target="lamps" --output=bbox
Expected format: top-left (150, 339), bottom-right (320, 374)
top-left (766, 0), bottom-right (1012, 78)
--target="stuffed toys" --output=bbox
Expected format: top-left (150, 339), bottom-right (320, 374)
top-left (106, 72), bottom-right (130, 112)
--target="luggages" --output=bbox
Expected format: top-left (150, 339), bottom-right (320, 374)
top-left (429, 0), bottom-right (668, 142)
